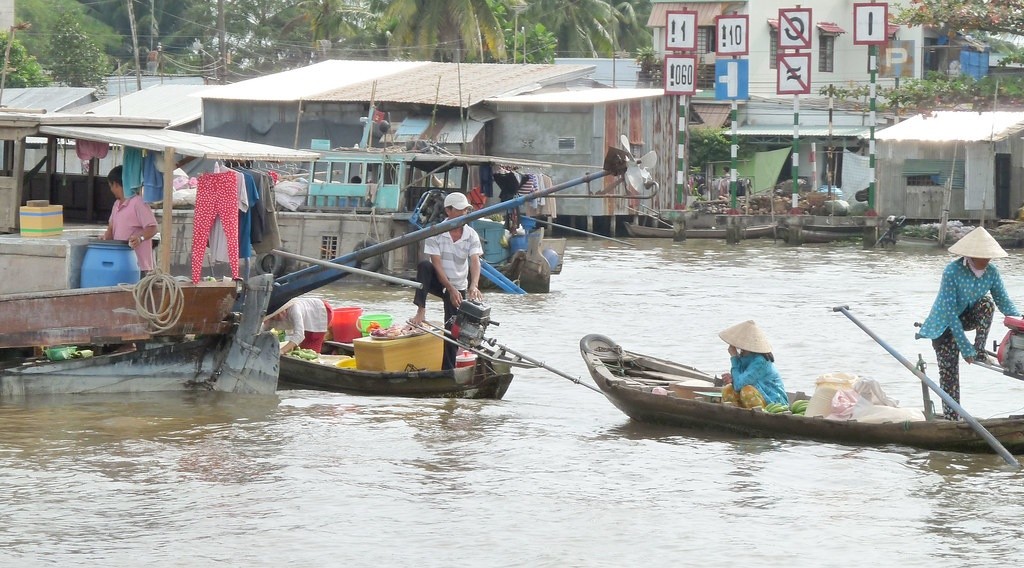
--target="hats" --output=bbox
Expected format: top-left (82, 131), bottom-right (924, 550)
top-left (443, 192), bottom-right (473, 210)
top-left (948, 226), bottom-right (1009, 258)
top-left (718, 320), bottom-right (773, 353)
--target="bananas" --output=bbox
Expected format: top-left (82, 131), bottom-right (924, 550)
top-left (764, 399), bottom-right (808, 416)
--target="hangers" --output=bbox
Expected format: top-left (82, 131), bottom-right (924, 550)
top-left (201, 159), bottom-right (310, 177)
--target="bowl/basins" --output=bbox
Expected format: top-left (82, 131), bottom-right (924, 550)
top-left (335, 358), bottom-right (356, 369)
top-left (543, 248), bottom-right (558, 269)
top-left (519, 215), bottom-right (536, 229)
top-left (44, 347), bottom-right (78, 361)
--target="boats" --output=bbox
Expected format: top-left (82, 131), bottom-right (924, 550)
top-left (579, 332), bottom-right (1024, 457)
top-left (279, 300), bottom-right (602, 402)
top-left (622, 220), bottom-right (779, 240)
top-left (781, 216), bottom-right (907, 244)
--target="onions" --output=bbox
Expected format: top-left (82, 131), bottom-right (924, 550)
top-left (371, 323), bottom-right (430, 337)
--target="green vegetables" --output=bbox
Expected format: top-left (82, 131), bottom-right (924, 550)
top-left (278, 330), bottom-right (286, 341)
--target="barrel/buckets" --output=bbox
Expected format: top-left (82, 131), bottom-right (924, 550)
top-left (333, 307), bottom-right (362, 342)
top-left (80, 240), bottom-right (140, 288)
top-left (456, 350), bottom-right (478, 368)
top-left (508, 235), bottom-right (528, 256)
top-left (356, 315), bottom-right (393, 337)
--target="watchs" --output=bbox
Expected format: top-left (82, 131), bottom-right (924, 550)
top-left (139, 234), bottom-right (144, 243)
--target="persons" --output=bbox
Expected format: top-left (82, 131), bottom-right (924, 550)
top-left (350, 176), bottom-right (362, 183)
top-left (717, 320), bottom-right (789, 412)
top-left (919, 227), bottom-right (1024, 422)
top-left (722, 166), bottom-right (731, 179)
top-left (410, 192), bottom-right (484, 371)
top-left (99, 165), bottom-right (158, 350)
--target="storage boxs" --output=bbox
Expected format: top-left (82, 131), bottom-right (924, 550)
top-left (19, 205), bottom-right (64, 238)
top-left (352, 331), bottom-right (444, 372)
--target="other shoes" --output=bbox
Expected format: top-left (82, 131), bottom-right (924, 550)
top-left (975, 350), bottom-right (987, 361)
